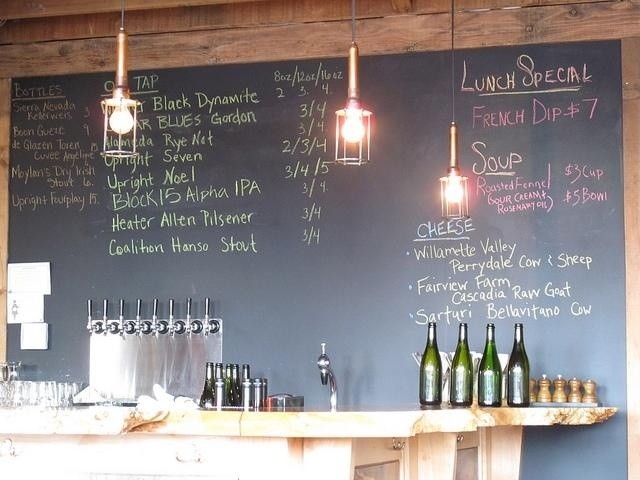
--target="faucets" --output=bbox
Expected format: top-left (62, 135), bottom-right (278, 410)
top-left (314, 343), bottom-right (337, 410)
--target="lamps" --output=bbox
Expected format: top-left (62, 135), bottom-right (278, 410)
top-left (99, 0), bottom-right (141, 160)
top-left (440, 0), bottom-right (470, 221)
top-left (334, 0), bottom-right (373, 166)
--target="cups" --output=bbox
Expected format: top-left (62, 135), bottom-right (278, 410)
top-left (0, 358), bottom-right (83, 407)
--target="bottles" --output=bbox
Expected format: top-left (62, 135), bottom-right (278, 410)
top-left (526, 374), bottom-right (598, 403)
top-left (418, 321), bottom-right (531, 408)
top-left (198, 360), bottom-right (269, 411)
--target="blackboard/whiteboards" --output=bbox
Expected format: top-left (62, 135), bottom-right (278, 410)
top-left (0, 0), bottom-right (639, 480)
